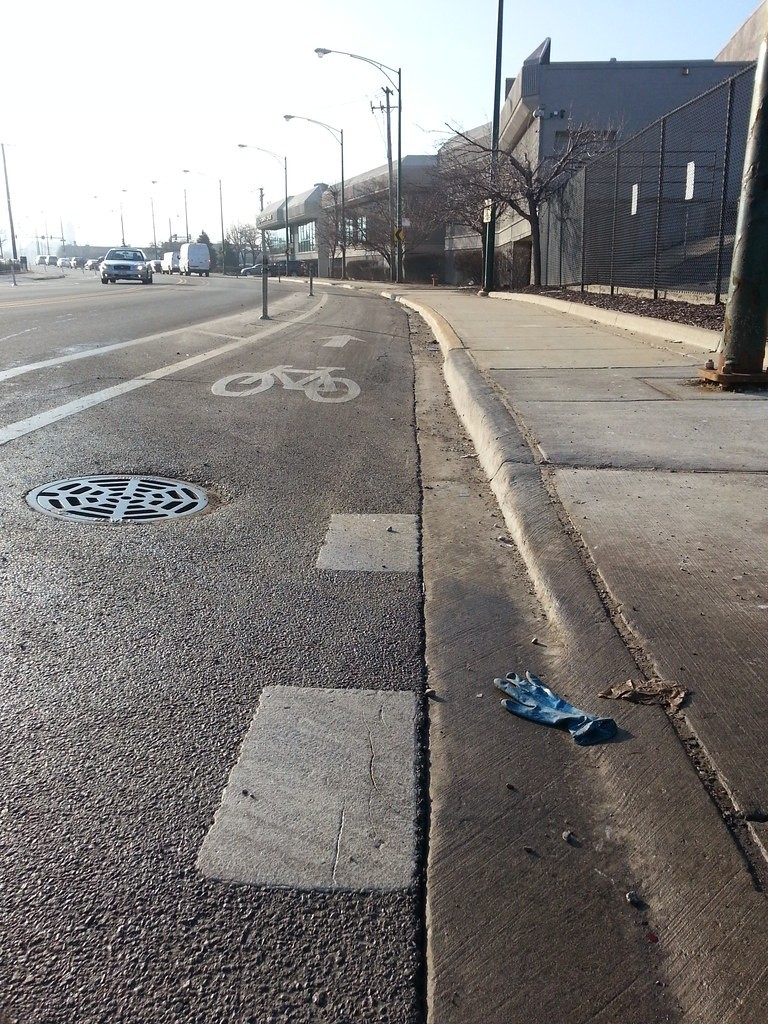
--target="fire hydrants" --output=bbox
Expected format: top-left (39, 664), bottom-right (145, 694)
top-left (431, 274), bottom-right (439, 286)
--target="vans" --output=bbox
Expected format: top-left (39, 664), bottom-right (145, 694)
top-left (270, 260), bottom-right (299, 277)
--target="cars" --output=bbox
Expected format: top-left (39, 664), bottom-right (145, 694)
top-left (35, 255), bottom-right (103, 271)
top-left (241, 263), bottom-right (272, 275)
top-left (152, 260), bottom-right (162, 273)
top-left (100, 247), bottom-right (153, 284)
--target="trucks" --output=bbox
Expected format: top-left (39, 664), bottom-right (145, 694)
top-left (161, 252), bottom-right (180, 275)
top-left (177, 242), bottom-right (210, 277)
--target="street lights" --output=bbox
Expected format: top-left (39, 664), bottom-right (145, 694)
top-left (284, 115), bottom-right (350, 281)
top-left (122, 190), bottom-right (157, 260)
top-left (238, 144), bottom-right (293, 276)
top-left (315, 48), bottom-right (403, 283)
top-left (152, 180), bottom-right (188, 242)
top-left (183, 170), bottom-right (228, 275)
top-left (94, 196), bottom-right (125, 245)
